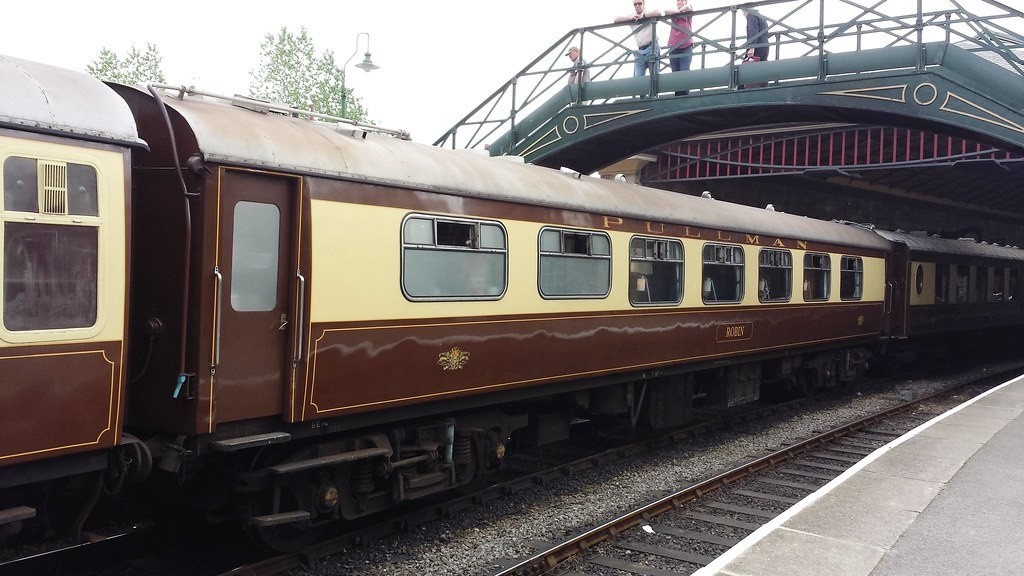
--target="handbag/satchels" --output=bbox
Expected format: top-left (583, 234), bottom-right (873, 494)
top-left (743, 56), bottom-right (768, 88)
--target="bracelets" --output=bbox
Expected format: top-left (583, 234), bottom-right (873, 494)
top-left (643, 13), bottom-right (646, 19)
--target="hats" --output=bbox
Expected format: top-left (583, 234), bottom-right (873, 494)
top-left (565, 47), bottom-right (579, 56)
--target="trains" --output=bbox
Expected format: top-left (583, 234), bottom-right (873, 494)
top-left (0, 54), bottom-right (1024, 571)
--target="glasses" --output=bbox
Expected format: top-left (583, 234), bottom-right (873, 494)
top-left (634, 1), bottom-right (643, 6)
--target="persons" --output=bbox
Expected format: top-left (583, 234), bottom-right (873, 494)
top-left (614, 0), bottom-right (661, 99)
top-left (665, 0), bottom-right (693, 96)
top-left (564, 47), bottom-right (590, 106)
top-left (736, 4), bottom-right (770, 91)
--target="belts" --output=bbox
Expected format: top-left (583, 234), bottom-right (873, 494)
top-left (639, 42), bottom-right (651, 49)
